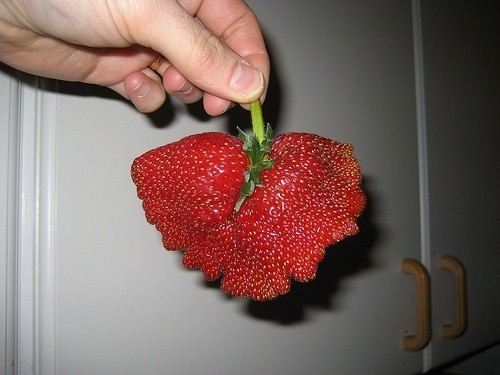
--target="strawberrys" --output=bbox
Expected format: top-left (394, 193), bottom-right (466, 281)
top-left (130, 131), bottom-right (367, 302)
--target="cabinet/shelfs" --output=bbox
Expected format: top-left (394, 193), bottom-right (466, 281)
top-left (42, 0), bottom-right (500, 375)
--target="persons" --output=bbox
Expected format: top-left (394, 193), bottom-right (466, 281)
top-left (1, 1), bottom-right (270, 116)
